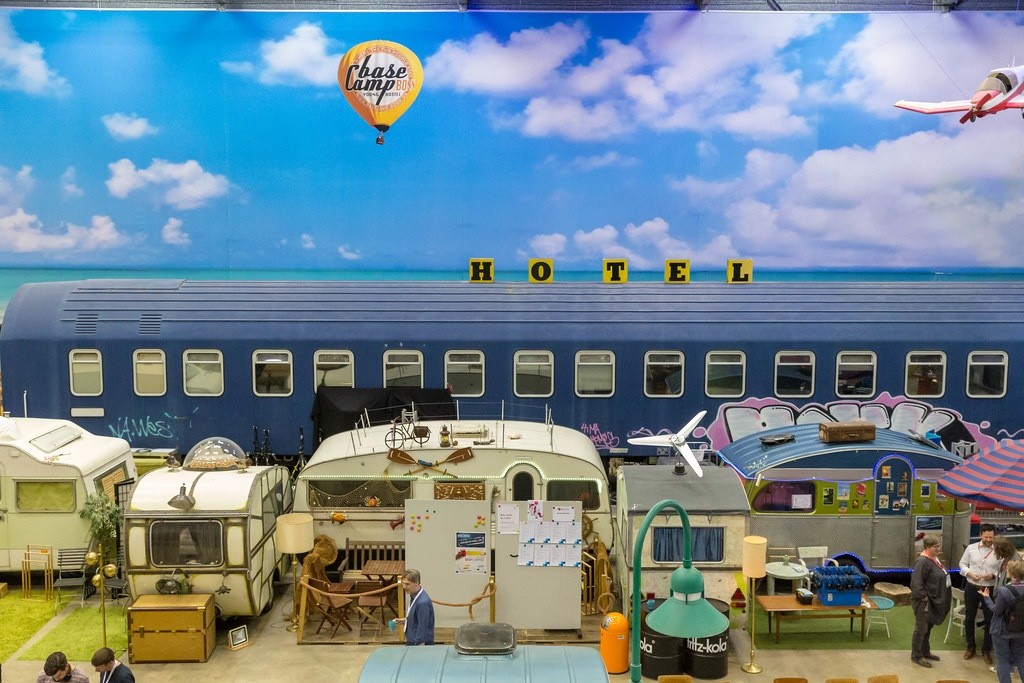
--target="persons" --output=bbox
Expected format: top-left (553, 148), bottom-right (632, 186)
top-left (392, 568), bottom-right (435, 646)
top-left (91, 647), bottom-right (135, 683)
top-left (35, 651), bottom-right (89, 683)
top-left (910, 534), bottom-right (952, 668)
top-left (977, 560), bottom-right (1024, 683)
top-left (959, 523), bottom-right (1004, 665)
top-left (988, 537), bottom-right (1024, 677)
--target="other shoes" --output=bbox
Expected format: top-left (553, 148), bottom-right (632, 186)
top-left (964, 647), bottom-right (975, 659)
top-left (983, 651), bottom-right (993, 664)
top-left (925, 652), bottom-right (941, 661)
top-left (911, 658), bottom-right (932, 668)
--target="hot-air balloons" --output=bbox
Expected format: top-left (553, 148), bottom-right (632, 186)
top-left (338, 39), bottom-right (425, 145)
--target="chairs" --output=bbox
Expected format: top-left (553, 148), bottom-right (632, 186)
top-left (305, 556), bottom-right (354, 639)
top-left (866, 597), bottom-right (894, 639)
top-left (943, 587), bottom-right (985, 645)
top-left (53, 548), bottom-right (89, 609)
top-left (797, 546), bottom-right (839, 592)
top-left (355, 581), bottom-right (398, 625)
top-left (96, 546), bottom-right (132, 616)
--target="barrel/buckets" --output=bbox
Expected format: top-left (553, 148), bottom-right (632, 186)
top-left (684, 597), bottom-right (731, 680)
top-left (640, 598), bottom-right (686, 682)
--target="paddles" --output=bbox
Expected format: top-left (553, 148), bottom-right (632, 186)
top-left (403, 447), bottom-right (474, 476)
top-left (387, 448), bottom-right (458, 479)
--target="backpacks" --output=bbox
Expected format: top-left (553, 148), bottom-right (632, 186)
top-left (1003, 585), bottom-right (1024, 633)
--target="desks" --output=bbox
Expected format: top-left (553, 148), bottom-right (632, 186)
top-left (361, 560), bottom-right (406, 623)
top-left (753, 594), bottom-right (879, 643)
top-left (766, 561), bottom-right (809, 617)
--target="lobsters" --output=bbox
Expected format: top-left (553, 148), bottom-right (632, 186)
top-left (389, 513), bottom-right (405, 531)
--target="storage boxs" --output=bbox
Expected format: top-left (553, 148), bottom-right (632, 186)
top-left (812, 566), bottom-right (871, 606)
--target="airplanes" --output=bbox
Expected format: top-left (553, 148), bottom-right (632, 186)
top-left (894, 65), bottom-right (1024, 124)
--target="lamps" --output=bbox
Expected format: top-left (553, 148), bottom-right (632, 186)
top-left (168, 483), bottom-right (195, 510)
top-left (275, 512), bottom-right (314, 633)
top-left (224, 625), bottom-right (254, 652)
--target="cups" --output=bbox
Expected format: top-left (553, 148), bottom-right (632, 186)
top-left (388, 620), bottom-right (396, 633)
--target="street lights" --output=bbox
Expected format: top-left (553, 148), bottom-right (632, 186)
top-left (630, 499), bottom-right (730, 683)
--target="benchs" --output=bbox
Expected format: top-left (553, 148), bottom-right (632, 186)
top-left (337, 538), bottom-right (405, 596)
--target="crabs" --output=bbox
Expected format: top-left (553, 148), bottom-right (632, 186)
top-left (364, 495), bottom-right (381, 507)
top-left (328, 511), bottom-right (347, 526)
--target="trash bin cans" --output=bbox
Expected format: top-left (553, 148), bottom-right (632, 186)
top-left (599, 612), bottom-right (630, 674)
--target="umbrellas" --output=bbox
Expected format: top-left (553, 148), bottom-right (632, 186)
top-left (937, 438), bottom-right (1024, 511)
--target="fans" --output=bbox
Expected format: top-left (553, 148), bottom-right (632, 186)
top-left (627, 411), bottom-right (711, 478)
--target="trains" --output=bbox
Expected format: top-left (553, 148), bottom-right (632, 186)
top-left (0, 256), bottom-right (1024, 450)
